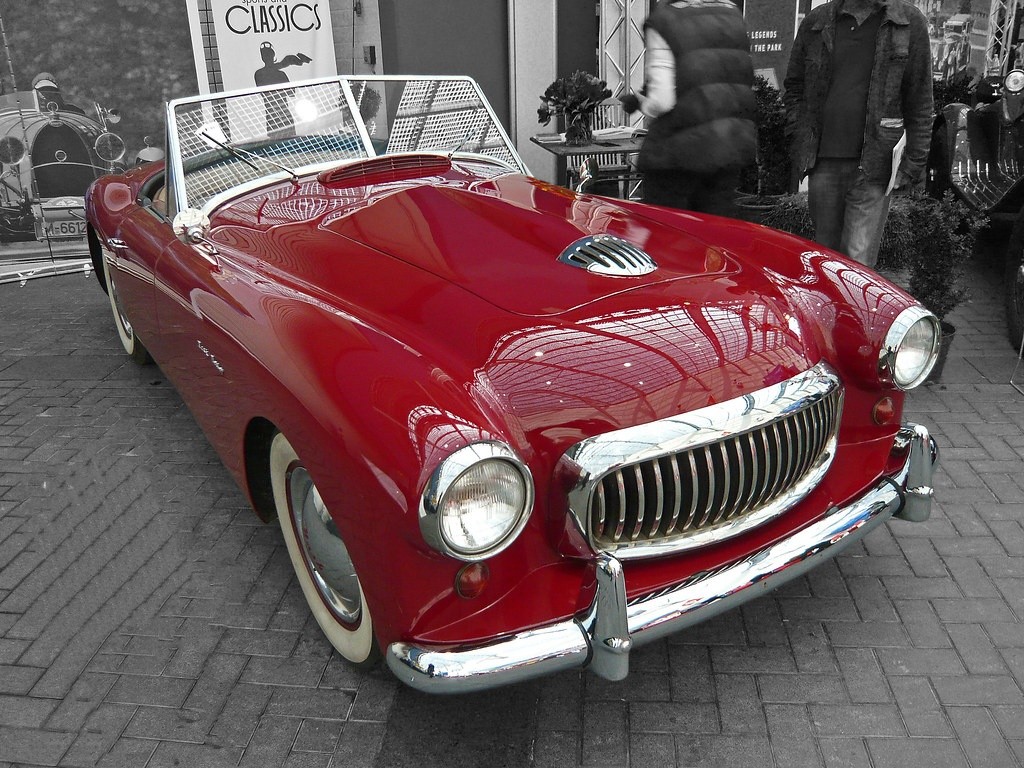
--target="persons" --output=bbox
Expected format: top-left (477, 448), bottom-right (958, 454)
top-left (782, 0), bottom-right (934, 270)
top-left (618, 0), bottom-right (760, 217)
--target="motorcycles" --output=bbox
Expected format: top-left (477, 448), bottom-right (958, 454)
top-left (926, 68), bottom-right (1024, 359)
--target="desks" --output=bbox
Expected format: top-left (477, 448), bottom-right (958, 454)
top-left (530, 134), bottom-right (647, 199)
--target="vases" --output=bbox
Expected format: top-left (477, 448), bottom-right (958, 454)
top-left (565, 114), bottom-right (591, 146)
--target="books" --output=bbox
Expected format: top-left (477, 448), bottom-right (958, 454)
top-left (536, 126), bottom-right (649, 143)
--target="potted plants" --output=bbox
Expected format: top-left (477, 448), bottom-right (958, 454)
top-left (730, 74), bottom-right (793, 224)
top-left (901, 167), bottom-right (976, 387)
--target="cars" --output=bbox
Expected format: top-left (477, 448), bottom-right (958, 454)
top-left (84, 76), bottom-right (944, 696)
top-left (0, 89), bottom-right (129, 241)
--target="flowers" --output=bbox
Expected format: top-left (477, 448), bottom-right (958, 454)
top-left (536, 69), bottom-right (613, 127)
